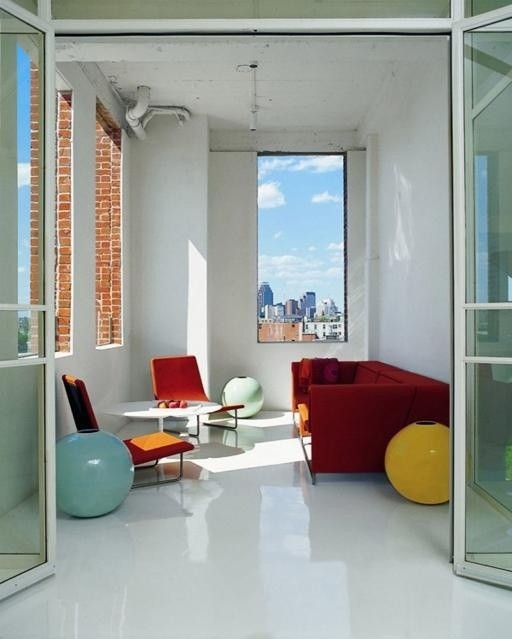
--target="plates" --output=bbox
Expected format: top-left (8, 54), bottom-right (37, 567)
top-left (150, 402), bottom-right (203, 412)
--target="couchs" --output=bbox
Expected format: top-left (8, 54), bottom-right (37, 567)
top-left (289, 361), bottom-right (449, 487)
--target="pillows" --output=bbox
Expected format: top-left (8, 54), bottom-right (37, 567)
top-left (297, 357), bottom-right (341, 393)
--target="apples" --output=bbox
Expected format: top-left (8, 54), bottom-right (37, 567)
top-left (159, 400), bottom-right (187, 408)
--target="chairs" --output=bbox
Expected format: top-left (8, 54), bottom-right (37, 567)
top-left (61, 353), bottom-right (246, 490)
